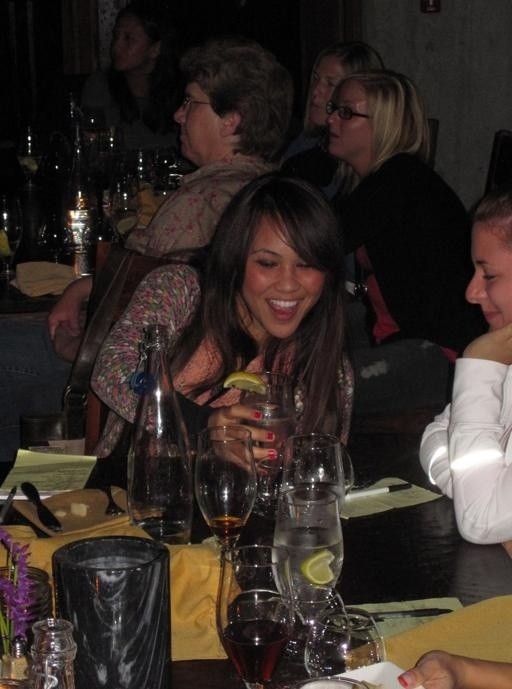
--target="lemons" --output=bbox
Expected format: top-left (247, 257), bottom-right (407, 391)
top-left (221, 371), bottom-right (269, 397)
top-left (303, 549), bottom-right (338, 585)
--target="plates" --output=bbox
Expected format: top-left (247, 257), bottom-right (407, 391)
top-left (13, 486), bottom-right (162, 537)
top-left (300, 659), bottom-right (426, 689)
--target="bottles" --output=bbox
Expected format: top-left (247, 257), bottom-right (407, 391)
top-left (60, 122), bottom-right (98, 255)
top-left (31, 617), bottom-right (78, 689)
top-left (126, 324), bottom-right (193, 546)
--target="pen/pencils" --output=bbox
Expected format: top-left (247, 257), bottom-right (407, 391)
top-left (344, 483), bottom-right (411, 502)
top-left (2, 486), bottom-right (16, 523)
top-left (370, 608), bottom-right (454, 621)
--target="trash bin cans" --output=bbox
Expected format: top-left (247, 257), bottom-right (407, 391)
top-left (54, 535), bottom-right (173, 689)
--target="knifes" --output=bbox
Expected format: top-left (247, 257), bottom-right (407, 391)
top-left (21, 481), bottom-right (64, 536)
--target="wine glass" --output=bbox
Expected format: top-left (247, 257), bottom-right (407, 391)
top-left (239, 372), bottom-right (297, 516)
top-left (196, 425), bottom-right (257, 582)
top-left (0, 196), bottom-right (23, 278)
top-left (272, 487), bottom-right (346, 654)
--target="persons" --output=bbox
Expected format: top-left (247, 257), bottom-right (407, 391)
top-left (415, 183), bottom-right (511, 570)
top-left (310, 66), bottom-right (498, 352)
top-left (85, 169), bottom-right (363, 503)
top-left (43, 39), bottom-right (301, 366)
top-left (269, 42), bottom-right (388, 182)
top-left (108, 4), bottom-right (198, 138)
top-left (397, 649), bottom-right (511, 688)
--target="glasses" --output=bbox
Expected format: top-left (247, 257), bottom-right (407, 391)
top-left (324, 97), bottom-right (373, 122)
top-left (179, 93), bottom-right (219, 110)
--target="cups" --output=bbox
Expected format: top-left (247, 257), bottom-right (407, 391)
top-left (216, 545), bottom-right (294, 688)
top-left (304, 607), bottom-right (385, 677)
top-left (282, 433), bottom-right (346, 516)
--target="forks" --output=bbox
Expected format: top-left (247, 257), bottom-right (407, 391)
top-left (97, 480), bottom-right (126, 516)
